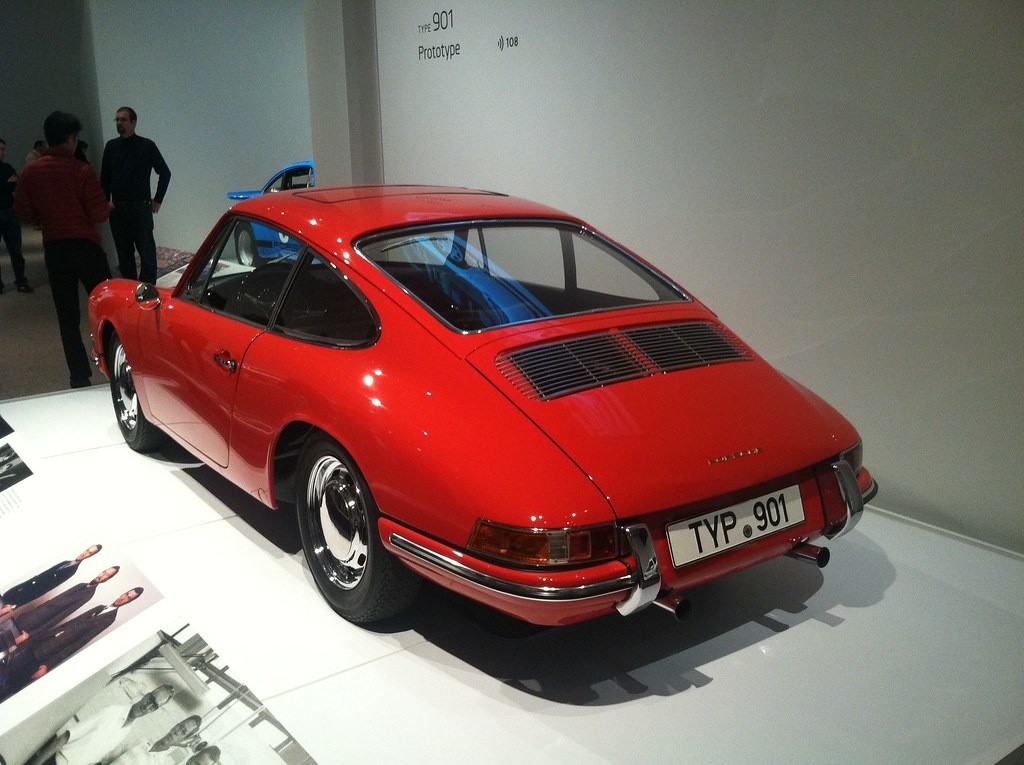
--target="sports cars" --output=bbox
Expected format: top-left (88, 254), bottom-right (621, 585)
top-left (87, 183), bottom-right (879, 628)
top-left (228, 161), bottom-right (316, 267)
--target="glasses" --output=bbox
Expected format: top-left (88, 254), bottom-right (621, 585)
top-left (114, 118), bottom-right (131, 123)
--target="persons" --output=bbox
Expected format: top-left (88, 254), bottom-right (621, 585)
top-left (100, 106), bottom-right (171, 285)
top-left (74, 139), bottom-right (88, 163)
top-left (15, 111), bottom-right (114, 389)
top-left (0, 545), bottom-right (144, 700)
top-left (0, 452), bottom-right (13, 474)
top-left (34, 685), bottom-right (221, 765)
top-left (26, 140), bottom-right (46, 164)
top-left (0, 139), bottom-right (34, 294)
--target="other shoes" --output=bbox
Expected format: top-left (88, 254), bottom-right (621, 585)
top-left (17, 285), bottom-right (33, 293)
top-left (69, 376), bottom-right (91, 388)
top-left (33, 223), bottom-right (42, 230)
top-left (0, 287), bottom-right (4, 296)
